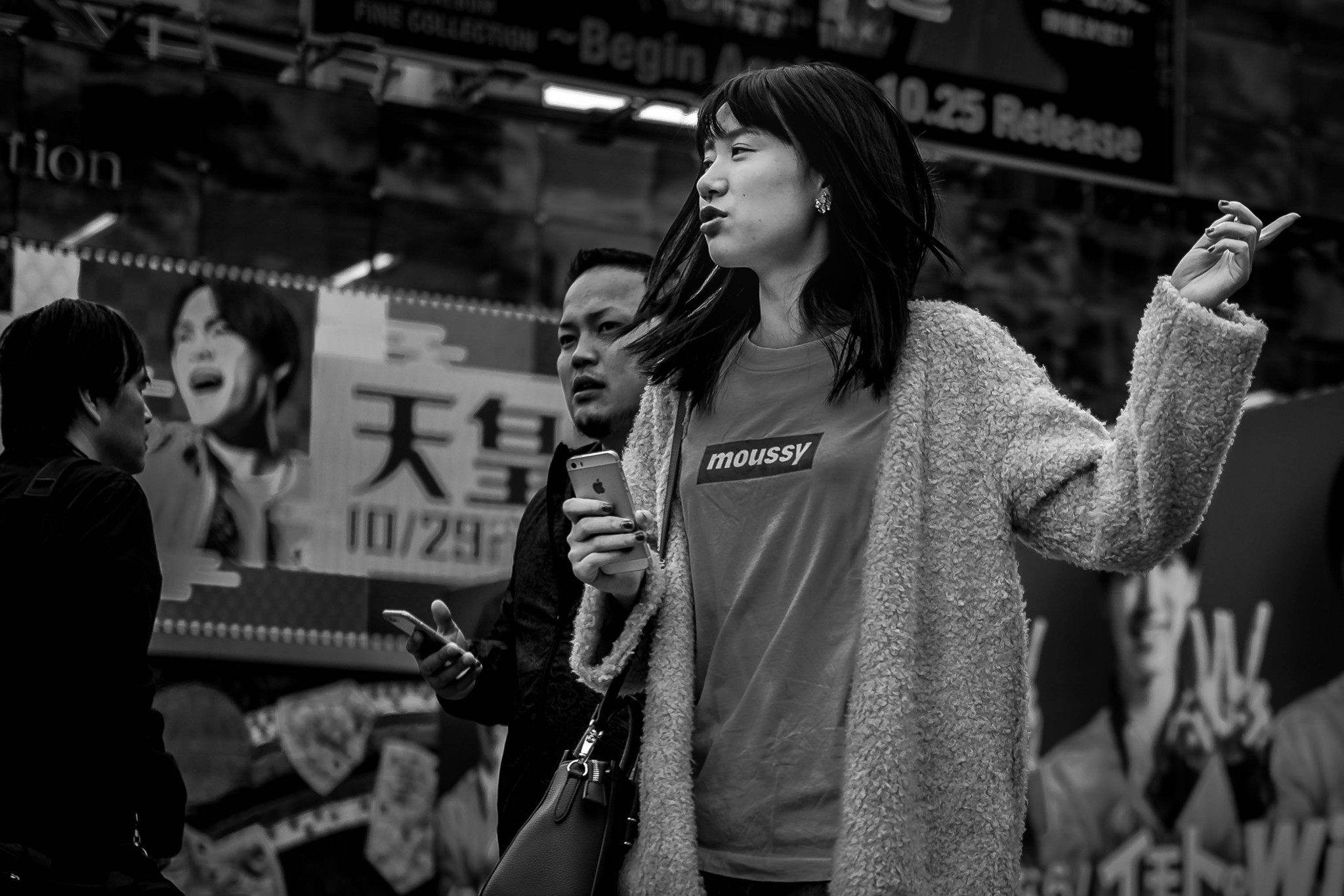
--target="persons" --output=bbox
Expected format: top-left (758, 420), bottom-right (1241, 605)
top-left (0, 295), bottom-right (192, 895)
top-left (561, 64), bottom-right (1302, 895)
top-left (404, 248), bottom-right (683, 859)
top-left (1026, 520), bottom-right (1344, 896)
top-left (139, 282), bottom-right (312, 573)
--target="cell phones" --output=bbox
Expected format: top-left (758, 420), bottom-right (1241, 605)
top-left (566, 450), bottom-right (653, 575)
top-left (383, 610), bottom-right (483, 680)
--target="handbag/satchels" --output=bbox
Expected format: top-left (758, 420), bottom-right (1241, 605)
top-left (477, 695), bottom-right (642, 896)
